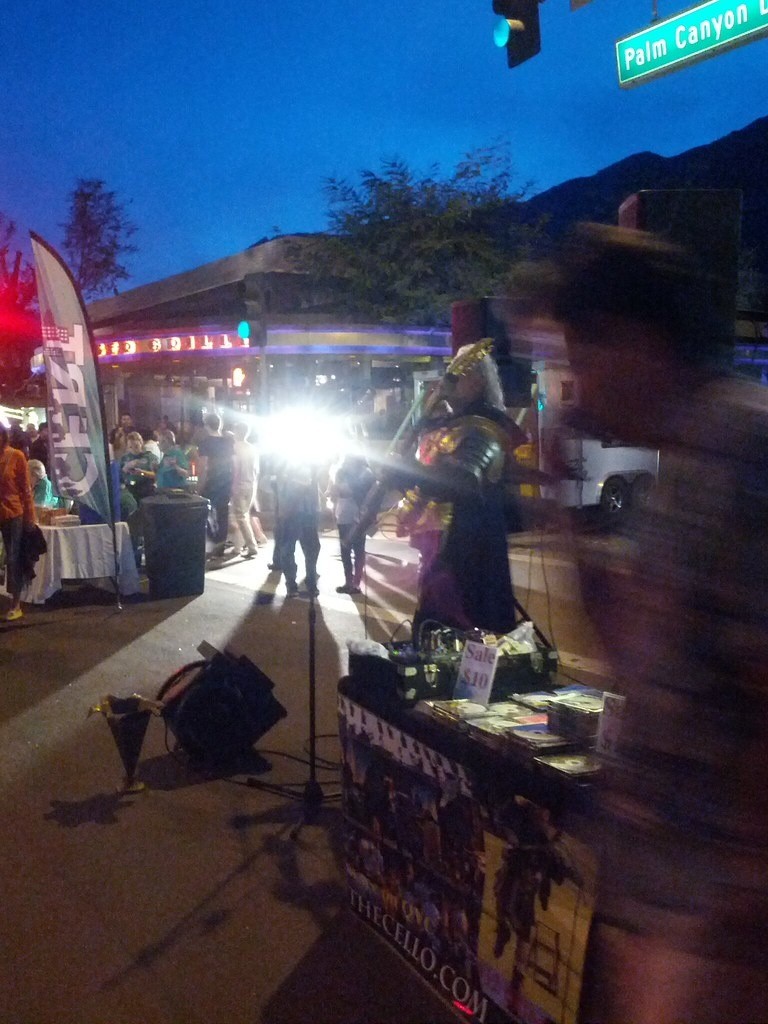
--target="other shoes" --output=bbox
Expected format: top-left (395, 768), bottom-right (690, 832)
top-left (299, 590), bottom-right (318, 599)
top-left (337, 584), bottom-right (360, 594)
top-left (0, 609), bottom-right (23, 620)
top-left (285, 589), bottom-right (300, 600)
top-left (269, 565), bottom-right (282, 570)
top-left (241, 550), bottom-right (257, 558)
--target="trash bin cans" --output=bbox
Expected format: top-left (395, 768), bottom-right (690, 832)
top-left (138, 492), bottom-right (211, 600)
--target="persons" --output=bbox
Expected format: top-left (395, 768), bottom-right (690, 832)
top-left (0, 410), bottom-right (321, 621)
top-left (362, 345), bottom-right (527, 648)
top-left (326, 440), bottom-right (374, 594)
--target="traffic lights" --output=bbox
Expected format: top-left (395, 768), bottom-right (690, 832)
top-left (490, 0), bottom-right (543, 71)
top-left (237, 274), bottom-right (267, 344)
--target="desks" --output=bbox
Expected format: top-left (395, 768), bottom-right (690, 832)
top-left (336, 659), bottom-right (619, 1024)
top-left (0, 523), bottom-right (142, 606)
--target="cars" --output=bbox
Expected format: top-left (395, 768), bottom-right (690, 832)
top-left (540, 420), bottom-right (662, 521)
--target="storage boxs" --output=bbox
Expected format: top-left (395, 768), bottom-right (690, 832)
top-left (547, 693), bottom-right (605, 744)
top-left (349, 639), bottom-right (451, 702)
top-left (470, 633), bottom-right (558, 692)
top-left (39, 506), bottom-right (81, 527)
